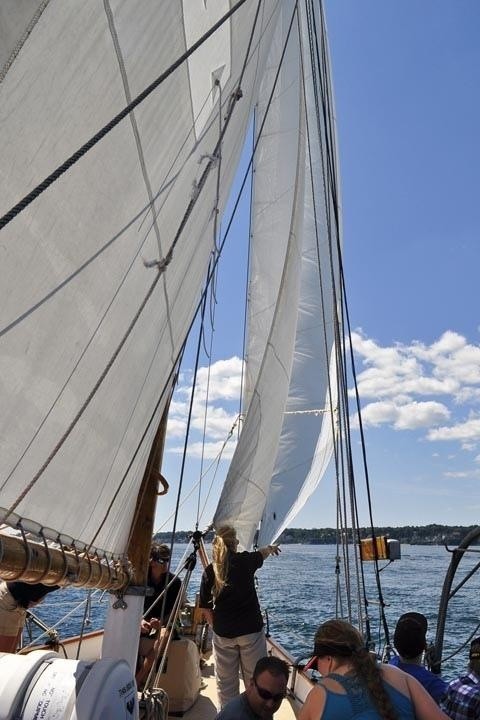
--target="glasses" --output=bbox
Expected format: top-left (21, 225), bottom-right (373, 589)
top-left (158, 557), bottom-right (170, 563)
top-left (254, 680), bottom-right (285, 701)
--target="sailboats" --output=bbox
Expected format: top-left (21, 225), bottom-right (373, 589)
top-left (2, 0), bottom-right (480, 718)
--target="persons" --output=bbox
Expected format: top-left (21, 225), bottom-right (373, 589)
top-left (437, 636), bottom-right (480, 720)
top-left (141, 541), bottom-right (181, 634)
top-left (296, 619), bottom-right (453, 720)
top-left (388, 612), bottom-right (448, 705)
top-left (212, 655), bottom-right (292, 720)
top-left (199, 526), bottom-right (282, 708)
top-left (0, 580), bottom-right (61, 653)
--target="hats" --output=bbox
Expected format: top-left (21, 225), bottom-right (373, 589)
top-left (394, 612), bottom-right (427, 659)
top-left (469, 637), bottom-right (480, 662)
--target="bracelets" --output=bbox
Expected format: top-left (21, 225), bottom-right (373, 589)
top-left (268, 545), bottom-right (274, 550)
